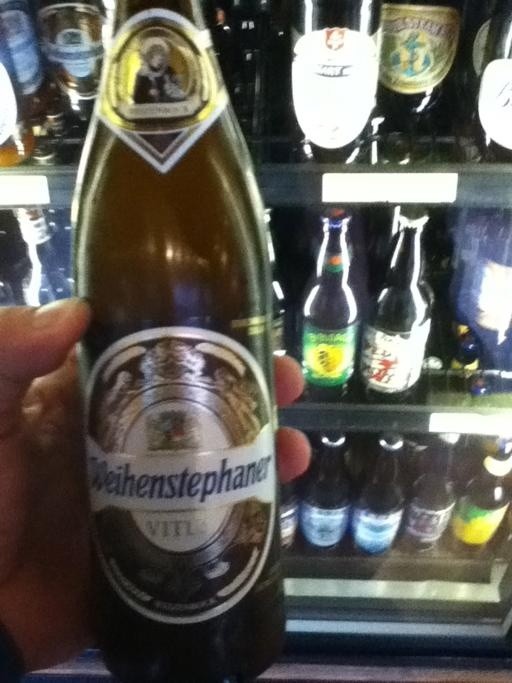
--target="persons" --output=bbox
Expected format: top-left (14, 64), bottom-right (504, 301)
top-left (0, 297), bottom-right (311, 681)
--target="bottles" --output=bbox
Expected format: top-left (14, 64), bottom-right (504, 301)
top-left (75, 0), bottom-right (288, 681)
top-left (279, 432), bottom-right (511, 555)
top-left (0, 0), bottom-right (103, 311)
top-left (264, 203), bottom-right (511, 406)
top-left (214, 2), bottom-right (512, 164)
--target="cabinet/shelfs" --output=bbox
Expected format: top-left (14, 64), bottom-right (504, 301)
top-left (1, 164), bottom-right (512, 668)
